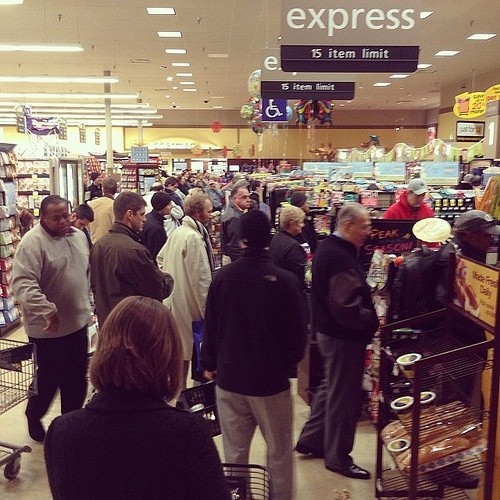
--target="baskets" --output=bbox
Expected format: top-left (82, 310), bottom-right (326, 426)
top-left (181, 380), bottom-right (221, 438)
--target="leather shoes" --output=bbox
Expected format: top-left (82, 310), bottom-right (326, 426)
top-left (326, 464), bottom-right (371, 478)
top-left (295, 448), bottom-right (324, 455)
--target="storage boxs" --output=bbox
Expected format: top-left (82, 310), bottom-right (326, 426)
top-left (177, 379), bottom-right (222, 438)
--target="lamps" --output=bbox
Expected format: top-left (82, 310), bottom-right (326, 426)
top-left (0, 74), bottom-right (163, 127)
top-left (0, 42), bottom-right (85, 52)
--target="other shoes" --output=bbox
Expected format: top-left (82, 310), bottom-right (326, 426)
top-left (28, 418), bottom-right (45, 442)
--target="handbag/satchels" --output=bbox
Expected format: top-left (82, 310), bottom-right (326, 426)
top-left (191, 319), bottom-right (204, 372)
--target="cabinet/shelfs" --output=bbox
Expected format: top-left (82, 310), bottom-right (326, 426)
top-left (246, 174), bottom-right (500, 500)
top-left (1, 139), bottom-right (158, 338)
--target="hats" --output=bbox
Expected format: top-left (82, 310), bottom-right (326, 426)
top-left (151, 192), bottom-right (172, 211)
top-left (408, 178), bottom-right (429, 196)
top-left (238, 209), bottom-right (271, 246)
top-left (249, 192), bottom-right (260, 202)
top-left (208, 179), bottom-right (216, 185)
top-left (91, 172), bottom-right (101, 182)
top-left (234, 173), bottom-right (245, 179)
top-left (290, 192), bottom-right (308, 208)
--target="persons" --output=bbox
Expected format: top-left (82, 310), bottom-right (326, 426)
top-left (12, 196), bottom-right (93, 443)
top-left (44, 296), bottom-right (233, 500)
top-left (383, 179), bottom-right (441, 252)
top-left (425, 210), bottom-right (500, 489)
top-left (198, 209), bottom-right (311, 500)
top-left (294, 202), bottom-right (378, 478)
top-left (67, 168), bottom-right (311, 389)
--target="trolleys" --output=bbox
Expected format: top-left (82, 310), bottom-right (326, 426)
top-left (218, 461), bottom-right (274, 500)
top-left (0, 337), bottom-right (41, 482)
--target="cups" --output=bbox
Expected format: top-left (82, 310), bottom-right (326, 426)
top-left (418, 392), bottom-right (437, 415)
top-left (397, 353), bottom-right (422, 379)
top-left (391, 396), bottom-right (414, 422)
top-left (388, 439), bottom-right (409, 468)
